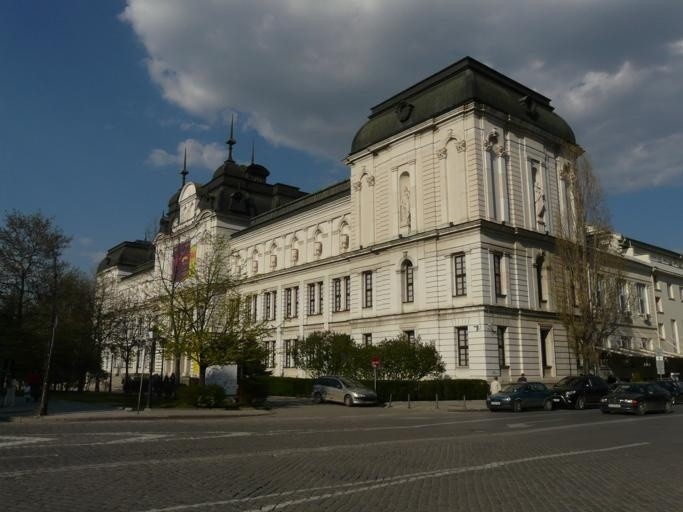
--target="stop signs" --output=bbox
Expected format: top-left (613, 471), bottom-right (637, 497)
top-left (370, 356), bottom-right (380, 367)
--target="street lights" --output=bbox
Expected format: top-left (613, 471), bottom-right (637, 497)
top-left (145, 324), bottom-right (162, 412)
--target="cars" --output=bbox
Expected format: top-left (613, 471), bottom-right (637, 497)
top-left (306, 372), bottom-right (378, 408)
top-left (485, 371), bottom-right (683, 417)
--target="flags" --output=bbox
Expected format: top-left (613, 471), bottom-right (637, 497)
top-left (170, 239), bottom-right (191, 283)
top-left (189, 242), bottom-right (197, 277)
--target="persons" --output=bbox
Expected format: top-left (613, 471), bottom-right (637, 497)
top-left (516, 373), bottom-right (527, 382)
top-left (488, 375), bottom-right (501, 394)
